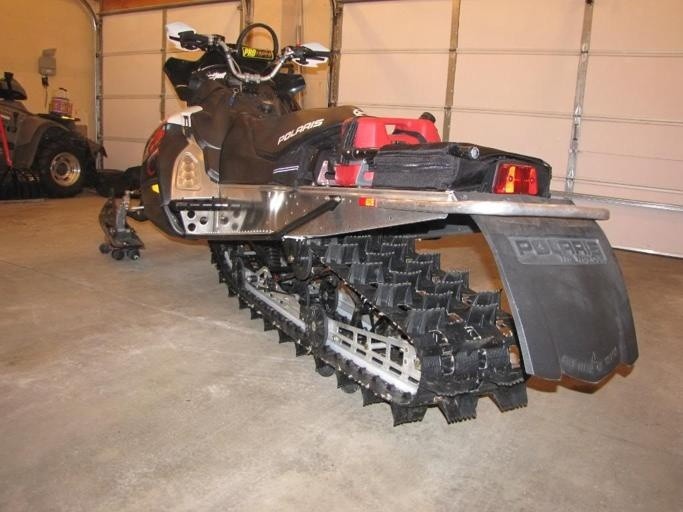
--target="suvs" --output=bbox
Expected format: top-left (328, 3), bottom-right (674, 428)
top-left (1, 73), bottom-right (106, 200)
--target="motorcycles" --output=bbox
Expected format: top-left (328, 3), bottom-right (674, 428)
top-left (98, 22), bottom-right (639, 427)
